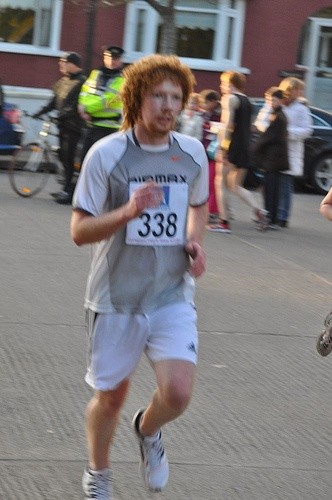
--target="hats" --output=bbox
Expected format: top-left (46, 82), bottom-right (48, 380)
top-left (60, 51), bottom-right (81, 67)
top-left (101, 45), bottom-right (124, 57)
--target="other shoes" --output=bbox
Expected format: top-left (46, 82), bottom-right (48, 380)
top-left (49, 192), bottom-right (72, 205)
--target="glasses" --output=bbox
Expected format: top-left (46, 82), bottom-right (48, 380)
top-left (57, 60), bottom-right (71, 64)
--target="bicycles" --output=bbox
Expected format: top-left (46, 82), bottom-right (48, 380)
top-left (9, 110), bottom-right (92, 198)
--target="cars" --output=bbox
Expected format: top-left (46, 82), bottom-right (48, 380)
top-left (215, 97), bottom-right (332, 196)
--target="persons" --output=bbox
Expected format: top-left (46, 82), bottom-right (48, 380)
top-left (33, 46), bottom-right (312, 233)
top-left (71, 55), bottom-right (210, 500)
top-left (317, 186), bottom-right (332, 357)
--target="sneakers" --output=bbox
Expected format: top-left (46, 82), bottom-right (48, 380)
top-left (205, 218), bottom-right (233, 233)
top-left (251, 208), bottom-right (271, 232)
top-left (81, 466), bottom-right (114, 500)
top-left (131, 406), bottom-right (170, 492)
top-left (315, 310), bottom-right (332, 357)
